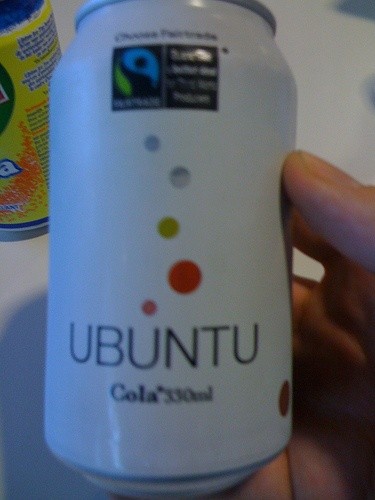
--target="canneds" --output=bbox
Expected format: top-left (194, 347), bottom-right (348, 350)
top-left (0, 0), bottom-right (63, 233)
top-left (43, 1), bottom-right (297, 500)
